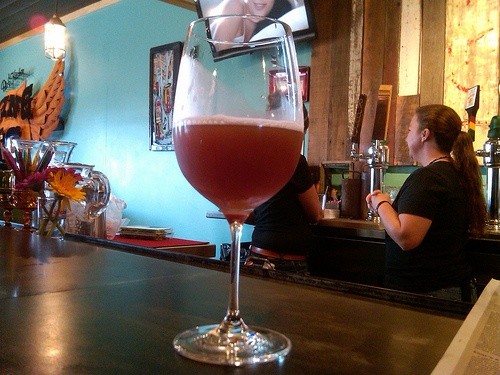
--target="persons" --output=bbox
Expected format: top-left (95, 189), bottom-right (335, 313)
top-left (203, 0), bottom-right (294, 52)
top-left (366, 104), bottom-right (479, 307)
top-left (249, 100), bottom-right (324, 279)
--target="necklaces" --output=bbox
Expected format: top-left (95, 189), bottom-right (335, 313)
top-left (425, 154), bottom-right (452, 168)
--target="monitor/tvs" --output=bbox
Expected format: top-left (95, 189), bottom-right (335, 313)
top-left (195, 0), bottom-right (318, 62)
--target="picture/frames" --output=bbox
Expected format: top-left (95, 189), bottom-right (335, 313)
top-left (267, 66), bottom-right (309, 107)
top-left (150, 41), bottom-right (182, 152)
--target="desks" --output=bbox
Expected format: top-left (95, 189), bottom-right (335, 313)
top-left (0, 221), bottom-right (499, 374)
top-left (112, 234), bottom-right (218, 258)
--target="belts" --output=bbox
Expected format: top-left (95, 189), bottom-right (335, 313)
top-left (249, 245), bottom-right (305, 260)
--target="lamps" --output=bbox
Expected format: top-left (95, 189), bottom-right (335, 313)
top-left (43, 0), bottom-right (70, 62)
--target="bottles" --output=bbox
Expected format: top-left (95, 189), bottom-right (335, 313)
top-left (483, 116), bottom-right (500, 220)
top-left (322, 186), bottom-right (331, 210)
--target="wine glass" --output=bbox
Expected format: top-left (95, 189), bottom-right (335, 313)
top-left (172, 14), bottom-right (304, 365)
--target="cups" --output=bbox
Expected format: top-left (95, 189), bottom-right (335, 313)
top-left (10, 138), bottom-right (77, 239)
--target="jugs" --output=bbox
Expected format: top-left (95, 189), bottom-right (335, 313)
top-left (55, 162), bottom-right (110, 240)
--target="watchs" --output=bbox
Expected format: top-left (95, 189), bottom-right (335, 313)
top-left (374, 200), bottom-right (391, 215)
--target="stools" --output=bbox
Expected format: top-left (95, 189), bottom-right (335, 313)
top-left (247, 245), bottom-right (306, 270)
